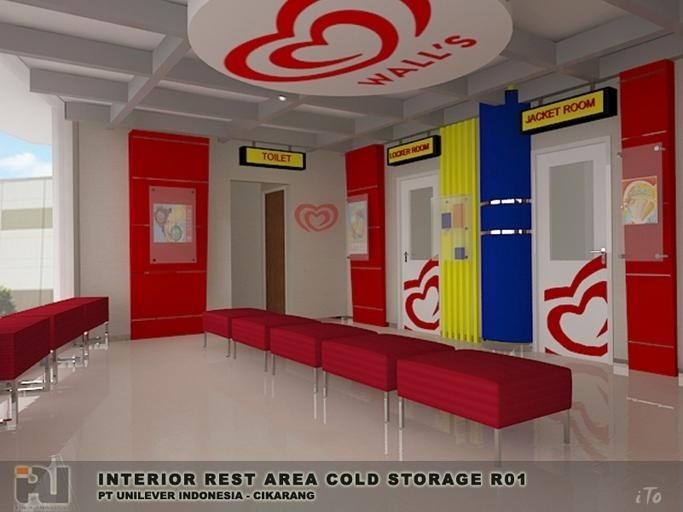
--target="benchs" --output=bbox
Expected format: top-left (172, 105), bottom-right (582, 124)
top-left (0, 297), bottom-right (109, 425)
top-left (204, 306), bottom-right (571, 467)
